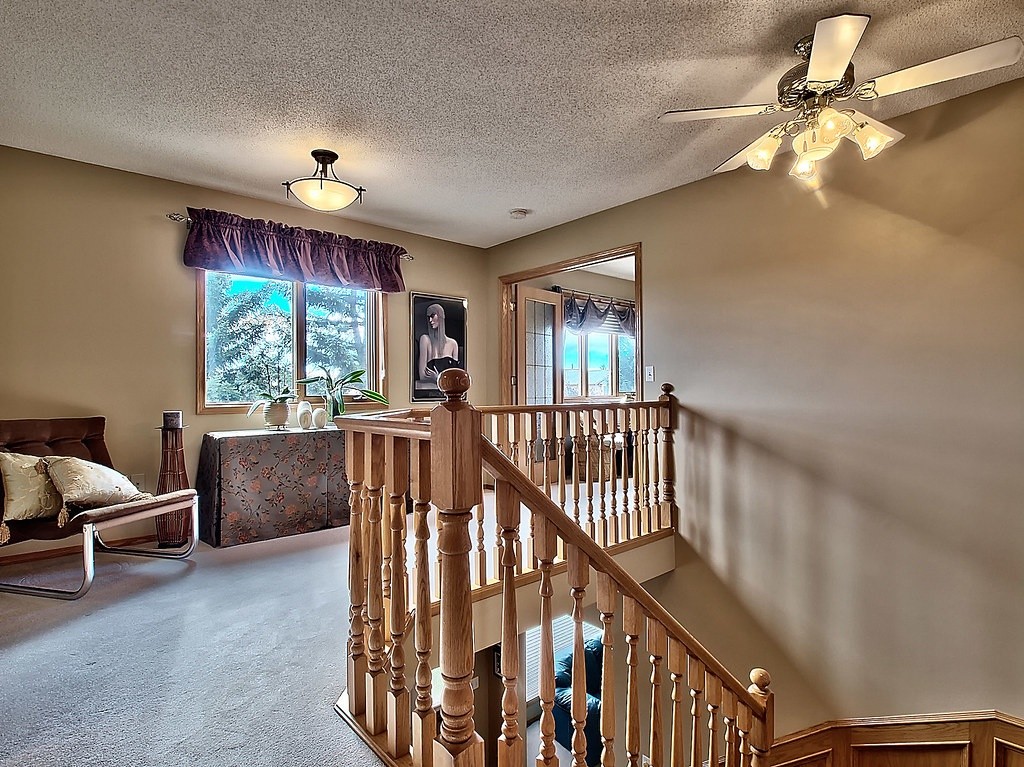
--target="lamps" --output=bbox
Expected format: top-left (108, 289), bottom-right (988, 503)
top-left (747, 108), bottom-right (894, 182)
top-left (280, 149), bottom-right (367, 213)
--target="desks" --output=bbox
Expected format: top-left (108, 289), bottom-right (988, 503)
top-left (198, 425), bottom-right (414, 548)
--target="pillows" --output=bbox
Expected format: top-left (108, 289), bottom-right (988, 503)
top-left (0, 451), bottom-right (65, 544)
top-left (35, 456), bottom-right (154, 527)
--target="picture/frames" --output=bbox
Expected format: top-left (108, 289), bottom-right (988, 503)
top-left (491, 649), bottom-right (503, 677)
top-left (408, 291), bottom-right (469, 403)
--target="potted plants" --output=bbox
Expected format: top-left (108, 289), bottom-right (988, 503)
top-left (245, 388), bottom-right (300, 426)
top-left (296, 364), bottom-right (390, 421)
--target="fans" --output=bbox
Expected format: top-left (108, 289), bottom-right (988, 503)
top-left (655, 12), bottom-right (1023, 172)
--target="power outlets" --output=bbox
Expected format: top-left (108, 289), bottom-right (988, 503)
top-left (131, 473), bottom-right (146, 492)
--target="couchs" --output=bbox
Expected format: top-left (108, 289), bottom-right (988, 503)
top-left (553, 635), bottom-right (604, 767)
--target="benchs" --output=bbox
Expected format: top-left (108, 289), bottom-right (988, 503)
top-left (0, 415), bottom-right (198, 600)
